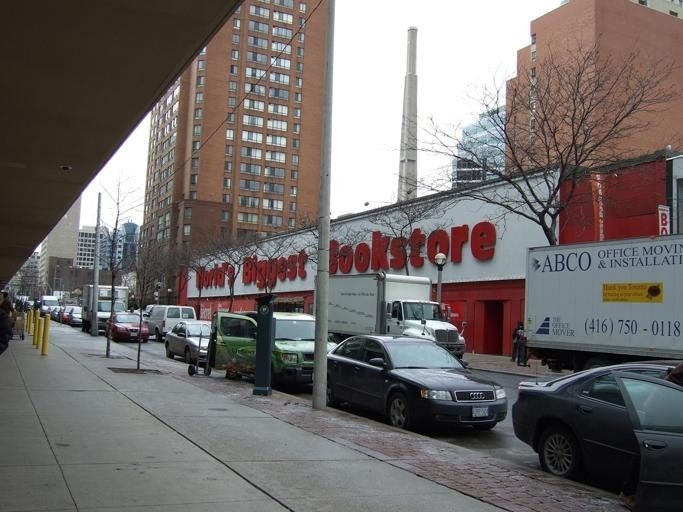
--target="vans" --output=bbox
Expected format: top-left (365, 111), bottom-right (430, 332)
top-left (214, 308), bottom-right (337, 389)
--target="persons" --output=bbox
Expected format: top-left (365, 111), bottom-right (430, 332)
top-left (0, 289), bottom-right (40, 355)
top-left (618, 360), bottom-right (682, 511)
top-left (128, 305), bottom-right (135, 314)
top-left (509, 320), bottom-right (523, 363)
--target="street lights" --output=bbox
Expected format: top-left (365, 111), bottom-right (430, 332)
top-left (434, 252), bottom-right (446, 302)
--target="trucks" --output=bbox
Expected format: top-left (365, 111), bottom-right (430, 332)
top-left (313, 273), bottom-right (468, 365)
top-left (522, 231), bottom-right (683, 372)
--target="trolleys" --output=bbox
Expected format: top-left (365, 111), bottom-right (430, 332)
top-left (187, 324), bottom-right (214, 377)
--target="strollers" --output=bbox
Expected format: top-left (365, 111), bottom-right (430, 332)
top-left (9, 311), bottom-right (25, 340)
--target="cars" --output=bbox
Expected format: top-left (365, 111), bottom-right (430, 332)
top-left (327, 334), bottom-right (509, 434)
top-left (164, 320), bottom-right (212, 364)
top-left (24, 283), bottom-right (196, 344)
top-left (510, 357), bottom-right (682, 512)
